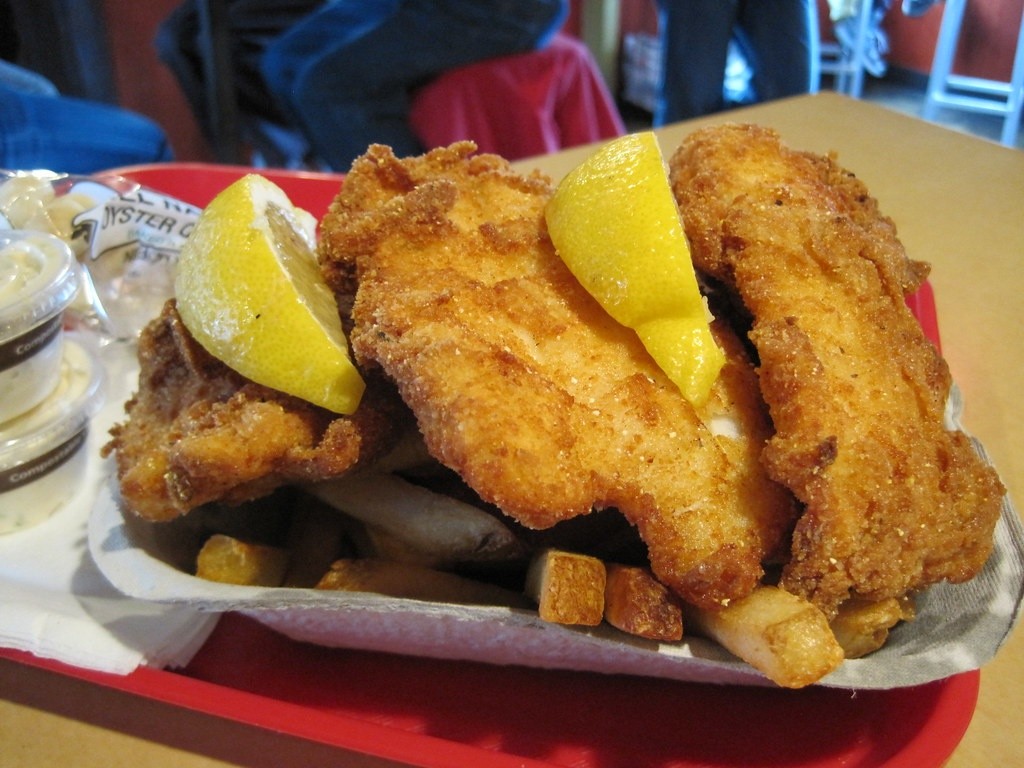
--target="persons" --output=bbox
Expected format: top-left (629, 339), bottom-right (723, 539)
top-left (655, 0), bottom-right (819, 133)
top-left (256, 0), bottom-right (568, 174)
top-left (0, 56), bottom-right (178, 176)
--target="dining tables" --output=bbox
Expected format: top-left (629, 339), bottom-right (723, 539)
top-left (0, 93), bottom-right (1024, 768)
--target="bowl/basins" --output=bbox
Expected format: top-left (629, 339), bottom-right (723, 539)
top-left (1, 333), bottom-right (109, 535)
top-left (0, 229), bottom-right (82, 424)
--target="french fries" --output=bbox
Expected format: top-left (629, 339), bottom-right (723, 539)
top-left (195, 424), bottom-right (917, 688)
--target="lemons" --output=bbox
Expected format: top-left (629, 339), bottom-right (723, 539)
top-left (175, 173), bottom-right (364, 419)
top-left (544, 129), bottom-right (726, 408)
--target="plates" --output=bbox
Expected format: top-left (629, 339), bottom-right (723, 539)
top-left (0, 164), bottom-right (978, 768)
